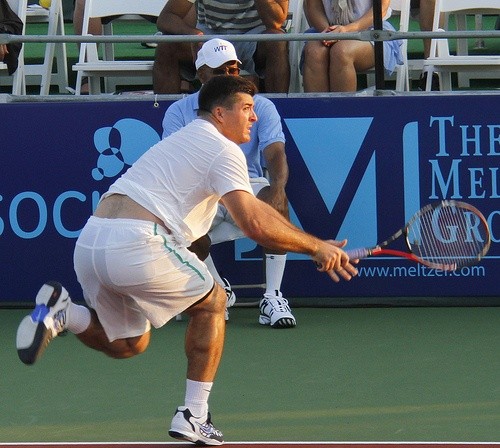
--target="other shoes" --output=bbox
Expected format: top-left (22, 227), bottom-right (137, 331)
top-left (81, 83), bottom-right (89, 94)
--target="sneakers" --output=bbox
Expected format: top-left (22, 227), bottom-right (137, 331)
top-left (15, 278), bottom-right (72, 367)
top-left (258, 289), bottom-right (297, 328)
top-left (220, 277), bottom-right (238, 321)
top-left (167, 405), bottom-right (225, 447)
top-left (141, 31), bottom-right (163, 48)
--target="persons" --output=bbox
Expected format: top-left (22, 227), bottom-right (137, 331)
top-left (16, 74), bottom-right (357, 445)
top-left (0, 0), bottom-right (448, 94)
top-left (161, 38), bottom-right (298, 328)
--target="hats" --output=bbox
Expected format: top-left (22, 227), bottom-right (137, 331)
top-left (195, 38), bottom-right (243, 71)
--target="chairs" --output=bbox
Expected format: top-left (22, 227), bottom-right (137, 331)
top-left (0, 0), bottom-right (500, 95)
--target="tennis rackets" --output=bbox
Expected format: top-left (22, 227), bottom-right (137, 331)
top-left (316, 199), bottom-right (491, 270)
